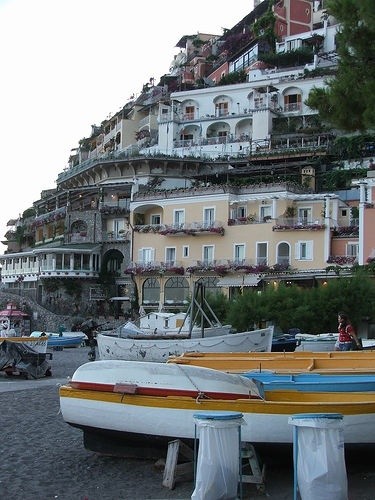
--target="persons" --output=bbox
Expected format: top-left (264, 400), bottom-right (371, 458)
top-left (336, 311), bottom-right (362, 352)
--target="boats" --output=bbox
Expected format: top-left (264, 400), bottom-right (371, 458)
top-left (59, 323), bottom-right (374, 444)
top-left (46, 336), bottom-right (85, 347)
top-left (0, 332), bottom-right (49, 356)
